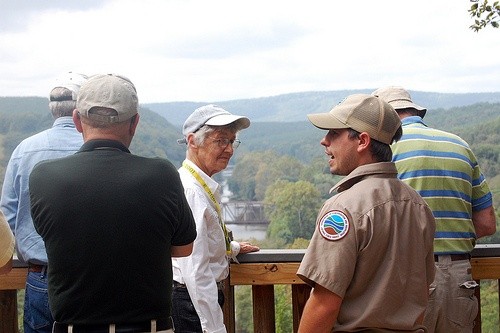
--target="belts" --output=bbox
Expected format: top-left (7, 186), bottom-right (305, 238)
top-left (172, 280), bottom-right (225, 290)
top-left (434, 253), bottom-right (471, 262)
top-left (28, 264), bottom-right (48, 273)
top-left (51, 316), bottom-right (174, 333)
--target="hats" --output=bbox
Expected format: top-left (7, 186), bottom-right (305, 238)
top-left (48, 71), bottom-right (88, 101)
top-left (307, 94), bottom-right (402, 145)
top-left (369, 86), bottom-right (427, 119)
top-left (76, 73), bottom-right (138, 122)
top-left (177, 105), bottom-right (250, 144)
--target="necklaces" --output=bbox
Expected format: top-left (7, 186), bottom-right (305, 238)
top-left (182, 161), bottom-right (240, 263)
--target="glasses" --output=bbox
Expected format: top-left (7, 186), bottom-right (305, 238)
top-left (207, 136), bottom-right (241, 149)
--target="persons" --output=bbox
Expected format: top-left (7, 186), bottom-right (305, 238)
top-left (0, 72), bottom-right (260, 333)
top-left (296, 85), bottom-right (496, 333)
